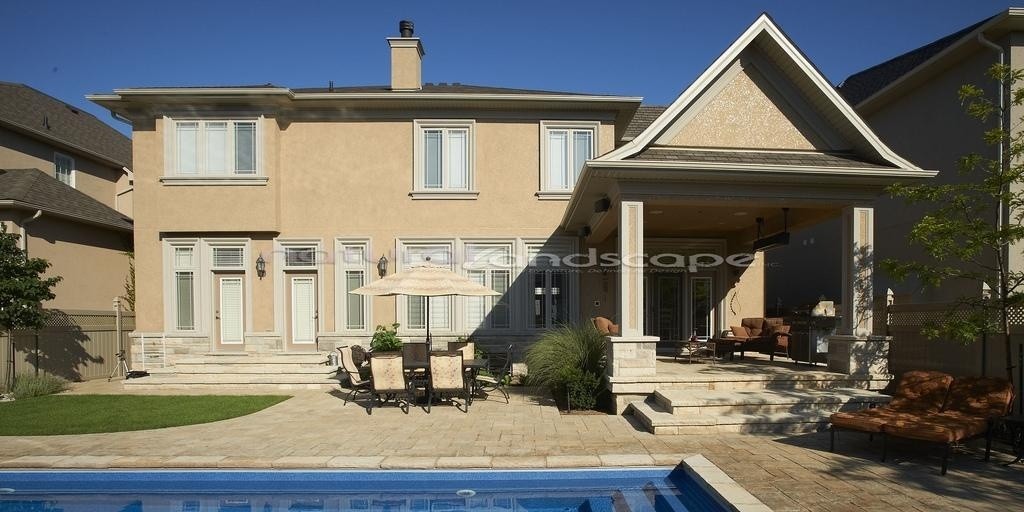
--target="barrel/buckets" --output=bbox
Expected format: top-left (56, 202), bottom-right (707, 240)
top-left (328, 354), bottom-right (338, 367)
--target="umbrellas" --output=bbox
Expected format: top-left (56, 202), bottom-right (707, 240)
top-left (348, 255), bottom-right (504, 350)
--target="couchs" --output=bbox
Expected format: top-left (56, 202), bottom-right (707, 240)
top-left (721, 329), bottom-right (793, 362)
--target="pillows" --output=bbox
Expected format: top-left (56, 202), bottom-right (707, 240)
top-left (608, 324), bottom-right (619, 336)
top-left (730, 326), bottom-right (748, 337)
top-left (764, 317), bottom-right (783, 335)
top-left (740, 317), bottom-right (765, 336)
top-left (595, 316), bottom-right (613, 333)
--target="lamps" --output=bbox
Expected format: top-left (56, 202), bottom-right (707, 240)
top-left (751, 207), bottom-right (790, 252)
top-left (255, 252), bottom-right (266, 281)
top-left (377, 252), bottom-right (389, 279)
top-left (592, 197), bottom-right (610, 213)
top-left (577, 225), bottom-right (591, 238)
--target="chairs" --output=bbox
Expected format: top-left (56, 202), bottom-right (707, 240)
top-left (589, 315), bottom-right (619, 336)
top-left (335, 340), bottom-right (514, 415)
top-left (826, 369), bottom-right (1017, 475)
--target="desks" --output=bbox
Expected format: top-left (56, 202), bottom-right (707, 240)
top-left (985, 412), bottom-right (1024, 467)
top-left (674, 340), bottom-right (717, 365)
top-left (708, 337), bottom-right (746, 361)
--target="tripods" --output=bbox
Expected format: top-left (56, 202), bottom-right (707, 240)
top-left (107, 307), bottom-right (130, 380)
